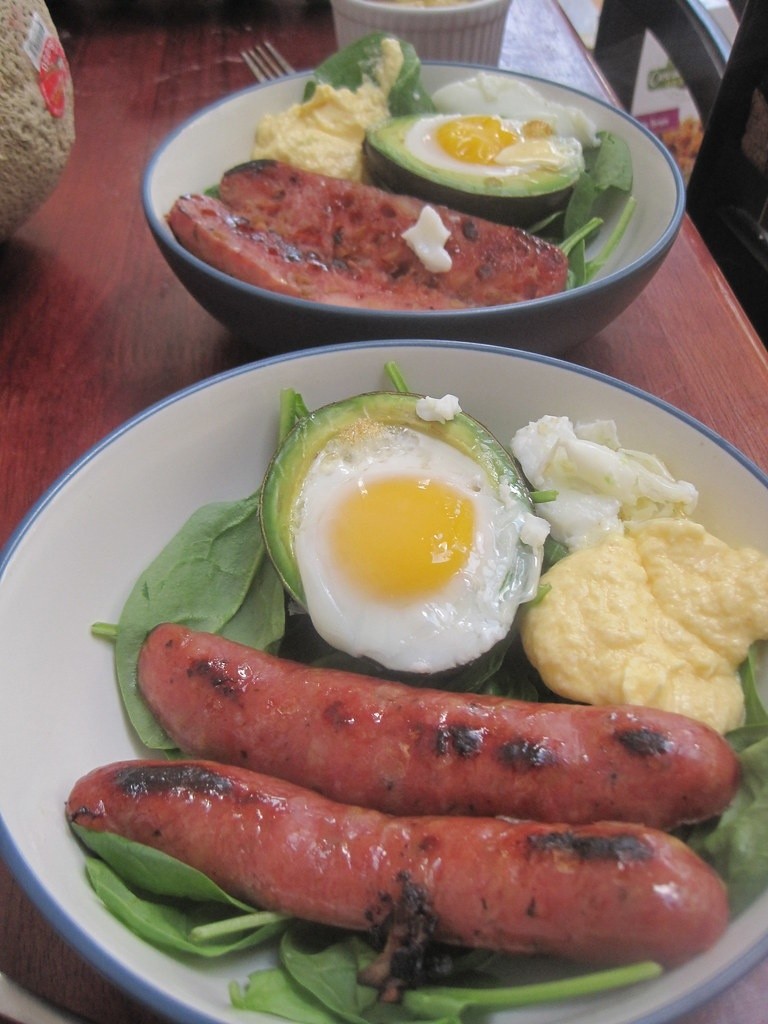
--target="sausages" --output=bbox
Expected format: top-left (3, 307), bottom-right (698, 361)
top-left (68, 614), bottom-right (741, 967)
top-left (178, 156), bottom-right (567, 310)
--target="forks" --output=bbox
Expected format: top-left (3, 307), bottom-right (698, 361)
top-left (240, 41), bottom-right (298, 85)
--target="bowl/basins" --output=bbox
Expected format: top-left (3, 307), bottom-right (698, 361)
top-left (143, 59), bottom-right (687, 363)
top-left (1, 335), bottom-right (767, 1022)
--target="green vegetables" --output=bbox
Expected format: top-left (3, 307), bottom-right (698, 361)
top-left (69, 364), bottom-right (768, 1024)
top-left (289, 36), bottom-right (640, 296)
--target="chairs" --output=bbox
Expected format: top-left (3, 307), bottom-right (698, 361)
top-left (592, 0), bottom-right (768, 247)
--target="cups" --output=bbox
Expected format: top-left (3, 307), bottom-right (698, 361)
top-left (330, 1), bottom-right (509, 70)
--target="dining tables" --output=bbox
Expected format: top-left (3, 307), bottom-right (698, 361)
top-left (2, 2), bottom-right (768, 1024)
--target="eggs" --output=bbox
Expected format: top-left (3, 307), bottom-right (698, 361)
top-left (297, 416), bottom-right (549, 673)
top-left (409, 108), bottom-right (581, 189)
